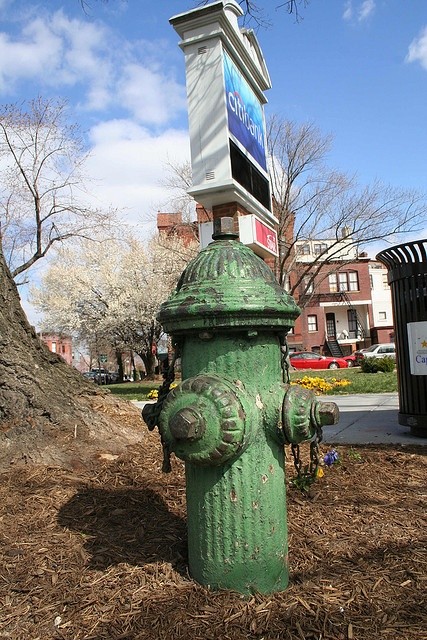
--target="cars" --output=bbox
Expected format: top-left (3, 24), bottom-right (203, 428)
top-left (288, 351), bottom-right (348, 369)
top-left (354, 344), bottom-right (395, 365)
top-left (342, 349), bottom-right (363, 367)
top-left (81, 367), bottom-right (119, 385)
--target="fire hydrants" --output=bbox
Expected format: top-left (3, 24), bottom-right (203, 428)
top-left (138, 213), bottom-right (341, 593)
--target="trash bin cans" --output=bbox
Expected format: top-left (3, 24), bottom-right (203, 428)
top-left (375, 239), bottom-right (427, 438)
